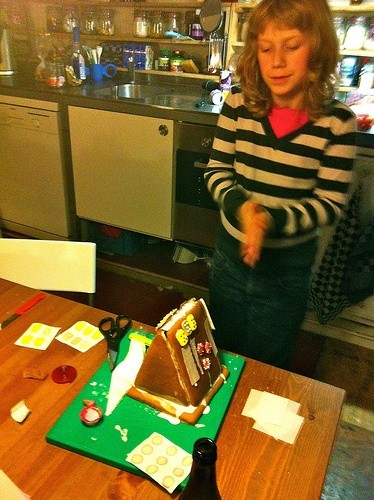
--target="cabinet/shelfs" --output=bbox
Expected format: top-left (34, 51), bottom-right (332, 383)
top-left (0, 0), bottom-right (374, 96)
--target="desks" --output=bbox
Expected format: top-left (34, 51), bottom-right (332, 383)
top-left (0, 272), bottom-right (348, 500)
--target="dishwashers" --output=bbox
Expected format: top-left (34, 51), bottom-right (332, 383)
top-left (1, 102), bottom-right (78, 241)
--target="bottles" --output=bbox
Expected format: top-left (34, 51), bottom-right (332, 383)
top-left (158, 49), bottom-right (185, 73)
top-left (333, 15), bottom-right (374, 52)
top-left (178, 437), bottom-right (222, 500)
top-left (69, 27), bottom-right (87, 81)
top-left (44, 5), bottom-right (115, 36)
top-left (132, 9), bottom-right (226, 40)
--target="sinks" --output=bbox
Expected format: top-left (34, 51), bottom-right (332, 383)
top-left (93, 81), bottom-right (171, 102)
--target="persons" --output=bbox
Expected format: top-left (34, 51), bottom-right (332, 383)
top-left (204, 0), bottom-right (356, 369)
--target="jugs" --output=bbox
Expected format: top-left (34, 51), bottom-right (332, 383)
top-left (0, 23), bottom-right (18, 76)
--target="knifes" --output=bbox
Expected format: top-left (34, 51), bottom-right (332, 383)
top-left (0, 293), bottom-right (45, 331)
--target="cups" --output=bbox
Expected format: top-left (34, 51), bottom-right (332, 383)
top-left (45, 62), bottom-right (65, 88)
top-left (88, 63), bottom-right (117, 83)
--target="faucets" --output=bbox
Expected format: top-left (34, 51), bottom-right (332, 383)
top-left (126, 61), bottom-right (143, 83)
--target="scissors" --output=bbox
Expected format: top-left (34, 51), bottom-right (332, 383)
top-left (99, 314), bottom-right (131, 372)
